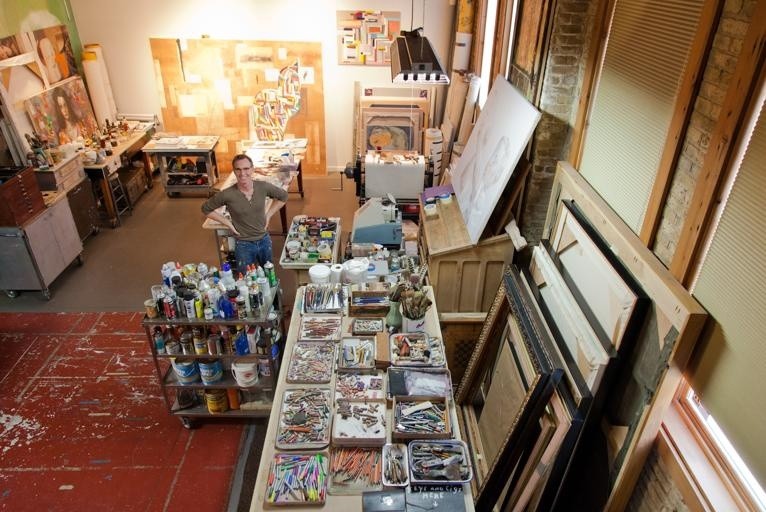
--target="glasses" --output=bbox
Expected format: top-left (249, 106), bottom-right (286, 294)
top-left (235, 167), bottom-right (250, 170)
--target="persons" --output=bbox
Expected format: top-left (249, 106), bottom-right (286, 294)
top-left (52, 86), bottom-right (90, 148)
top-left (200, 154), bottom-right (289, 274)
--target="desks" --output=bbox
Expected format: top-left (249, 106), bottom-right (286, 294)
top-left (277, 213), bottom-right (342, 280)
top-left (201, 140), bottom-right (309, 270)
top-left (248, 284), bottom-right (473, 510)
top-left (87, 122), bottom-right (156, 226)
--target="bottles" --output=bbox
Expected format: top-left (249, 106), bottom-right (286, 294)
top-left (152, 322), bottom-right (280, 358)
top-left (99, 115), bottom-right (130, 156)
top-left (150, 258), bottom-right (275, 320)
top-left (390, 252), bottom-right (401, 273)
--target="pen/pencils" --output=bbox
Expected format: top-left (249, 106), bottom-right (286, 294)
top-left (263, 284), bottom-right (470, 507)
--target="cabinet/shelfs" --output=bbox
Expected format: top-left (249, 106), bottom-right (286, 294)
top-left (0, 153), bottom-right (105, 299)
top-left (141, 277), bottom-right (284, 433)
top-left (143, 134), bottom-right (218, 199)
